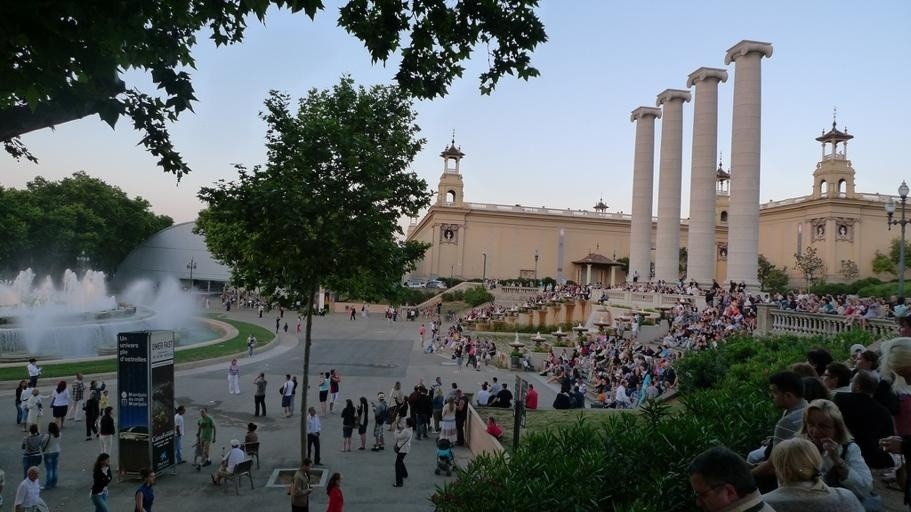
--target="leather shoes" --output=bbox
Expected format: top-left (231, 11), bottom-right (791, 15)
top-left (84, 437), bottom-right (92, 441)
top-left (96, 432), bottom-right (100, 438)
top-left (393, 481), bottom-right (403, 487)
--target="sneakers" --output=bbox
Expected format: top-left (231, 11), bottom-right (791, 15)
top-left (202, 460), bottom-right (211, 467)
top-left (210, 472), bottom-right (220, 485)
top-left (192, 463), bottom-right (196, 466)
top-left (280, 413), bottom-right (293, 419)
top-left (40, 486), bottom-right (48, 490)
top-left (339, 448), bottom-right (351, 453)
top-left (319, 414), bottom-right (326, 416)
top-left (196, 464), bottom-right (201, 470)
top-left (329, 411), bottom-right (336, 415)
top-left (20, 428), bottom-right (28, 432)
top-left (315, 462), bottom-right (323, 465)
top-left (414, 425), bottom-right (440, 440)
top-left (66, 416), bottom-right (81, 422)
top-left (371, 443), bottom-right (384, 452)
top-left (358, 446), bottom-right (365, 450)
top-left (177, 460), bottom-right (187, 464)
top-left (454, 439), bottom-right (464, 446)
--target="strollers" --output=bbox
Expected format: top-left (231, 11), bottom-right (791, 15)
top-left (434, 437), bottom-right (457, 476)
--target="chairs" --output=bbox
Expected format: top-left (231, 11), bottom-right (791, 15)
top-left (221, 459), bottom-right (254, 496)
top-left (243, 442), bottom-right (262, 468)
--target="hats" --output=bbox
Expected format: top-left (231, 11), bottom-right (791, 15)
top-left (29, 358), bottom-right (38, 363)
top-left (230, 439), bottom-right (240, 446)
top-left (248, 422), bottom-right (257, 431)
top-left (378, 394), bottom-right (384, 400)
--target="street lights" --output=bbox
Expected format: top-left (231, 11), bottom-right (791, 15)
top-left (885, 179), bottom-right (911, 299)
top-left (534, 249), bottom-right (539, 283)
top-left (75, 250), bottom-right (90, 279)
top-left (185, 256), bottom-right (197, 299)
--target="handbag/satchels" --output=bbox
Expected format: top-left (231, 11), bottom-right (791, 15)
top-left (355, 411), bottom-right (366, 426)
top-left (279, 386), bottom-right (286, 394)
top-left (353, 416), bottom-right (361, 429)
top-left (375, 411), bottom-right (387, 425)
top-left (35, 455), bottom-right (42, 464)
top-left (48, 397), bottom-right (56, 408)
top-left (393, 444), bottom-right (400, 453)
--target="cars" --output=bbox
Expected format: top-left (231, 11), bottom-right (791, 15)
top-left (406, 279), bottom-right (447, 290)
